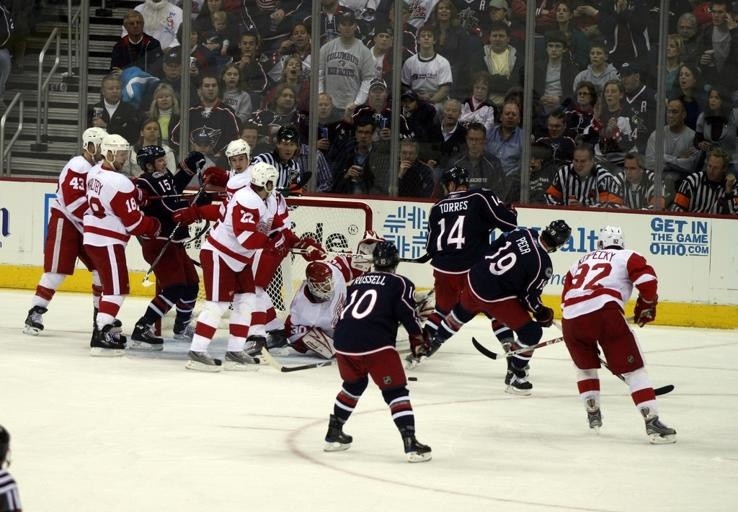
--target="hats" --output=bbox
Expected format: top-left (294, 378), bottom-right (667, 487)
top-left (336, 10), bottom-right (356, 26)
top-left (164, 50), bottom-right (179, 69)
top-left (486, 0), bottom-right (509, 11)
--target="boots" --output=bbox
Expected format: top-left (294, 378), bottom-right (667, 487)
top-left (587, 397), bottom-right (602, 430)
top-left (643, 406), bottom-right (678, 436)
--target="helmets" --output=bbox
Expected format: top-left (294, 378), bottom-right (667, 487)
top-left (543, 221), bottom-right (570, 252)
top-left (439, 168), bottom-right (471, 195)
top-left (374, 241), bottom-right (398, 270)
top-left (600, 226), bottom-right (626, 250)
top-left (81, 126), bottom-right (301, 198)
top-left (305, 262), bottom-right (334, 299)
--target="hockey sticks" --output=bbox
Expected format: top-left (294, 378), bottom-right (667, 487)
top-left (553, 320), bottom-right (674, 395)
top-left (261, 346), bottom-right (411, 371)
top-left (472, 312), bottom-right (654, 359)
top-left (143, 170), bottom-right (312, 200)
top-left (142, 176), bottom-right (213, 286)
top-left (287, 248), bottom-right (433, 263)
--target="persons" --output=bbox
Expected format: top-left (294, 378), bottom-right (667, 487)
top-left (24, 0), bottom-right (738, 397)
top-left (559, 225), bottom-right (679, 437)
top-left (324, 239), bottom-right (434, 455)
top-left (0, 427), bottom-right (24, 509)
top-left (0, 0), bottom-right (45, 96)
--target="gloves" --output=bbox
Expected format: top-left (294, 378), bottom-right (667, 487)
top-left (633, 295), bottom-right (658, 327)
top-left (535, 306), bottom-right (552, 326)
top-left (410, 332), bottom-right (431, 356)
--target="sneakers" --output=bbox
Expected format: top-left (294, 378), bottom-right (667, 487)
top-left (324, 414), bottom-right (352, 443)
top-left (24, 304), bottom-right (286, 366)
top-left (402, 429), bottom-right (432, 454)
top-left (506, 372), bottom-right (534, 390)
